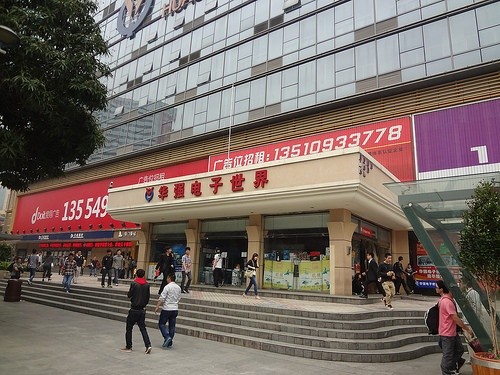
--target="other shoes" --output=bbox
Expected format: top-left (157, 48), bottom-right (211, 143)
top-left (381, 297), bottom-right (386, 306)
top-left (120, 348), bottom-right (132, 353)
top-left (145, 347), bottom-right (152, 354)
top-left (168, 343), bottom-right (173, 348)
top-left (242, 294), bottom-right (249, 298)
top-left (162, 337), bottom-right (172, 347)
top-left (255, 295), bottom-right (260, 299)
top-left (181, 289), bottom-right (189, 293)
top-left (64, 286), bottom-right (69, 293)
top-left (102, 284), bottom-right (105, 288)
top-left (27, 279), bottom-right (32, 286)
top-left (107, 285), bottom-right (113, 288)
top-left (387, 304), bottom-right (393, 310)
top-left (115, 283), bottom-right (119, 286)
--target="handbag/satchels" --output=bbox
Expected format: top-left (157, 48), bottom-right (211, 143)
top-left (11, 270), bottom-right (20, 278)
top-left (245, 269), bottom-right (253, 278)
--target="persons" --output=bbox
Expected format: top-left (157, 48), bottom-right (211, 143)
top-left (435, 281), bottom-right (474, 375)
top-left (121, 269), bottom-right (152, 354)
top-left (180, 247), bottom-right (192, 293)
top-left (212, 248), bottom-right (224, 286)
top-left (377, 253), bottom-right (396, 309)
top-left (10, 248), bottom-right (53, 285)
top-left (90, 255), bottom-right (100, 277)
top-left (242, 253), bottom-right (259, 299)
top-left (405, 263), bottom-right (418, 292)
top-left (72, 251), bottom-right (86, 284)
top-left (100, 249), bottom-right (113, 288)
top-left (62, 253), bottom-right (77, 293)
top-left (120, 256), bottom-right (135, 279)
top-left (112, 249), bottom-right (123, 285)
top-left (459, 278), bottom-right (483, 360)
top-left (154, 273), bottom-right (181, 347)
top-left (359, 252), bottom-right (385, 297)
top-left (395, 256), bottom-right (413, 295)
top-left (352, 271), bottom-right (366, 295)
top-left (155, 246), bottom-right (175, 295)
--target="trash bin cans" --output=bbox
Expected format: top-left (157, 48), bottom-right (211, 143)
top-left (4, 279), bottom-right (22, 302)
top-left (204, 267), bottom-right (214, 285)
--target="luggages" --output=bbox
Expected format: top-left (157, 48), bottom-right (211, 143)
top-left (3, 278), bottom-right (22, 302)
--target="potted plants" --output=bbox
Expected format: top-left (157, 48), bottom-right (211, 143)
top-left (454, 176), bottom-right (500, 375)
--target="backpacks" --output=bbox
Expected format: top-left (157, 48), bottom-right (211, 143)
top-left (5, 261), bottom-right (14, 271)
top-left (424, 299), bottom-right (439, 335)
